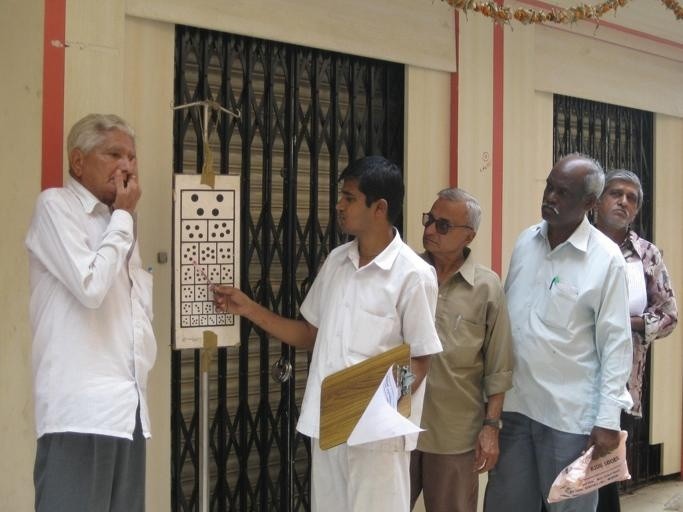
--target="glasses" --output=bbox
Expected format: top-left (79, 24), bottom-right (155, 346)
top-left (423, 212), bottom-right (473, 234)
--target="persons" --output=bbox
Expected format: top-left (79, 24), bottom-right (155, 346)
top-left (587, 167), bottom-right (679, 512)
top-left (401, 187), bottom-right (515, 512)
top-left (24, 109), bottom-right (158, 512)
top-left (210, 154), bottom-right (443, 512)
top-left (480, 151), bottom-right (635, 512)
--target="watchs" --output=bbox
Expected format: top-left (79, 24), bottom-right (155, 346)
top-left (482, 418), bottom-right (503, 430)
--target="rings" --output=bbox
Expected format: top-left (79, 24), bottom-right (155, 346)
top-left (128, 174), bottom-right (137, 180)
